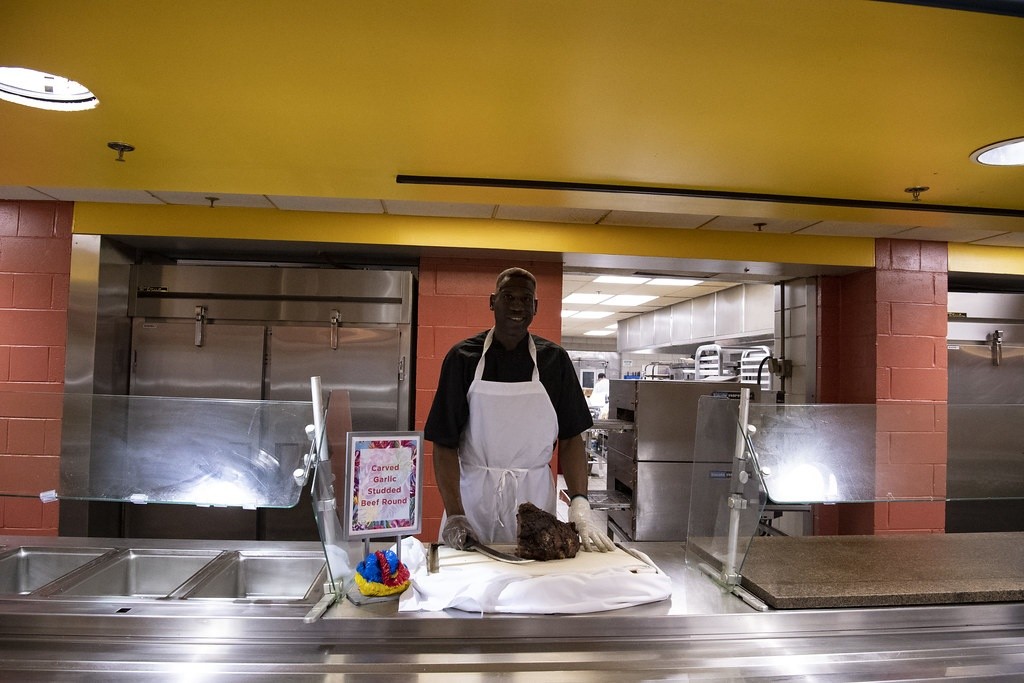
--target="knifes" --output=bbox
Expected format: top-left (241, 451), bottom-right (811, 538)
top-left (457, 536), bottom-right (534, 563)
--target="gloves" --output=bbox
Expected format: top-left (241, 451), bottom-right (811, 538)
top-left (568, 496), bottom-right (618, 553)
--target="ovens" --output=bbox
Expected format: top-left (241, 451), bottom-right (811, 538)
top-left (561, 379), bottom-right (761, 542)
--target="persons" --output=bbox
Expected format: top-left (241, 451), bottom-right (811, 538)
top-left (588, 373), bottom-right (609, 418)
top-left (424, 268), bottom-right (615, 553)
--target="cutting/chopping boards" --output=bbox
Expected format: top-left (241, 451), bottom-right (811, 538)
top-left (438, 544), bottom-right (646, 574)
